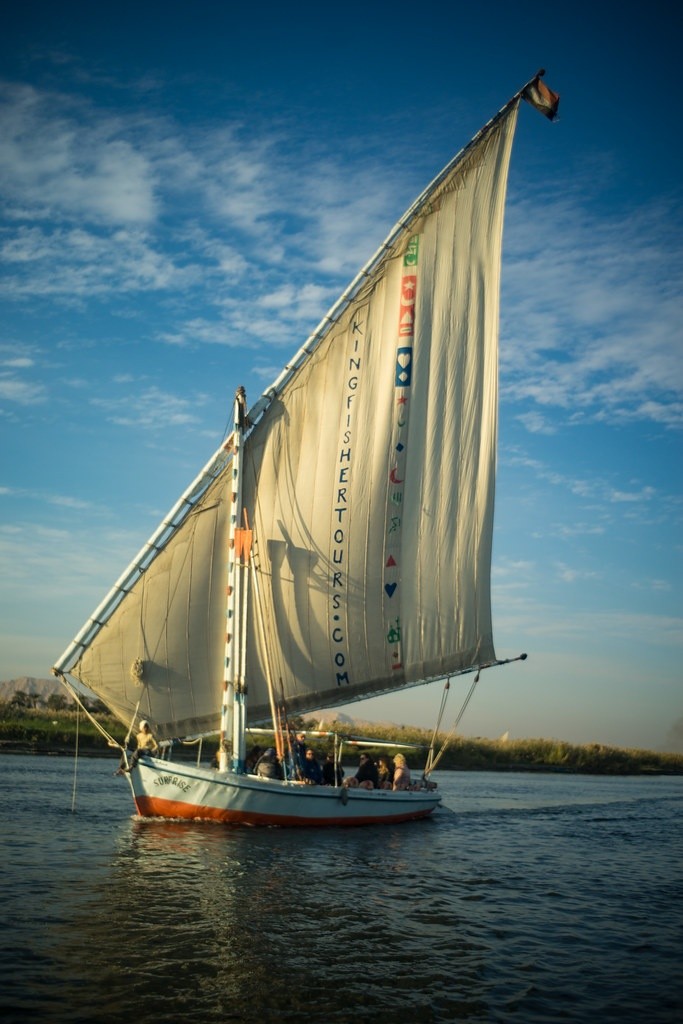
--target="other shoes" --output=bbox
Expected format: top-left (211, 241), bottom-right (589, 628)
top-left (119, 769), bottom-right (124, 775)
top-left (126, 769), bottom-right (132, 773)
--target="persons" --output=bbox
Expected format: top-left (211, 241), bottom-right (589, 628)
top-left (349, 753), bottom-right (378, 790)
top-left (216, 740), bottom-right (233, 770)
top-left (293, 735), bottom-right (307, 758)
top-left (322, 753), bottom-right (345, 787)
top-left (392, 753), bottom-right (409, 791)
top-left (299, 749), bottom-right (323, 785)
top-left (245, 746), bottom-right (261, 774)
top-left (253, 748), bottom-right (284, 780)
top-left (113, 719), bottom-right (158, 776)
top-left (377, 756), bottom-right (394, 790)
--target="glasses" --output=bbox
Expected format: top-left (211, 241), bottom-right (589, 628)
top-left (359, 758), bottom-right (364, 761)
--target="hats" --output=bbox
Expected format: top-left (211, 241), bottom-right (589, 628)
top-left (139, 719), bottom-right (150, 732)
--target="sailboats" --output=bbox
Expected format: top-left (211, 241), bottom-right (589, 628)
top-left (48, 68), bottom-right (561, 833)
